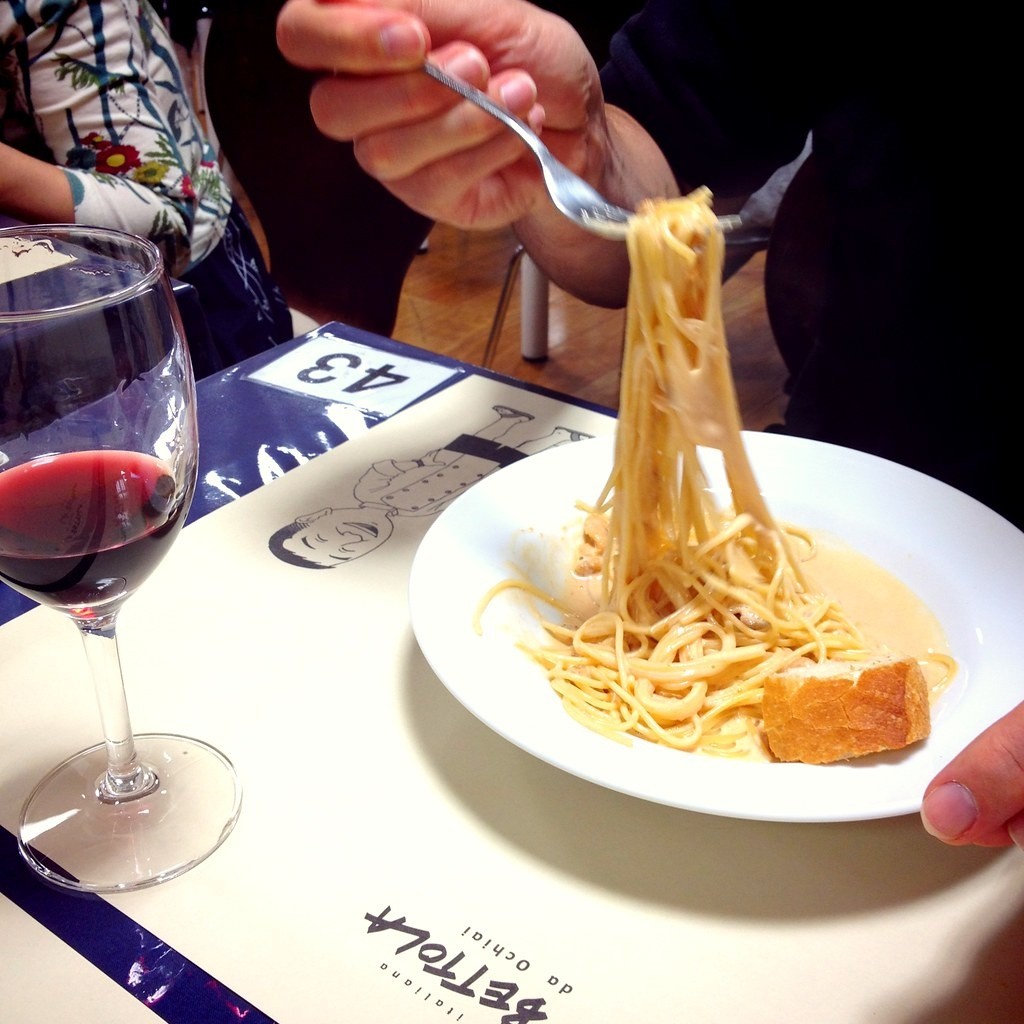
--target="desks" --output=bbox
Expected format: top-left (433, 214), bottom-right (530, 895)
top-left (1, 321), bottom-right (1024, 1024)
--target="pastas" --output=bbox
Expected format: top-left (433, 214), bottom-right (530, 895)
top-left (472, 186), bottom-right (958, 757)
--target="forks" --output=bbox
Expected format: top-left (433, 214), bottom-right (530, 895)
top-left (422, 60), bottom-right (742, 239)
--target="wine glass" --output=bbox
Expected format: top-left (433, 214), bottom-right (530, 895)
top-left (0, 222), bottom-right (244, 891)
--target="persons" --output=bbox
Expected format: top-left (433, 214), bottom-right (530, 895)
top-left (275, 2), bottom-right (1024, 847)
top-left (0, 0), bottom-right (294, 436)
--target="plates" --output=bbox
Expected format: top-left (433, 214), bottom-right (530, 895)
top-left (409, 431), bottom-right (1024, 822)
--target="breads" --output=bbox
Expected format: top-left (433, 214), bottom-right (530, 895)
top-left (761, 662), bottom-right (934, 767)
